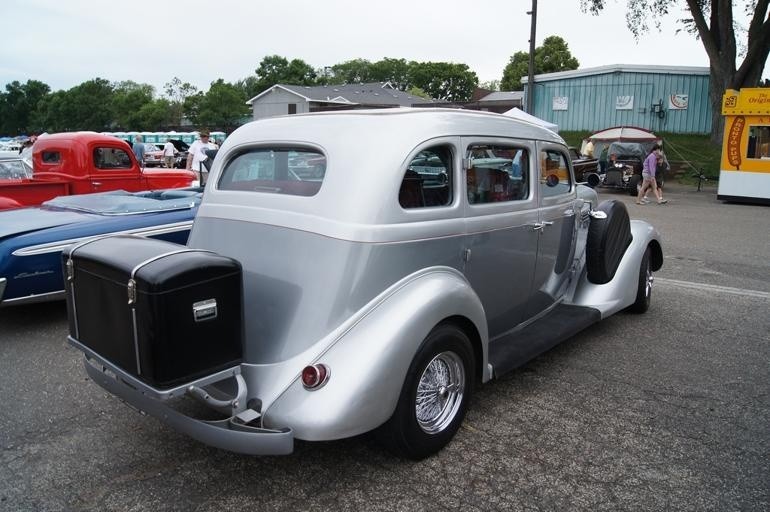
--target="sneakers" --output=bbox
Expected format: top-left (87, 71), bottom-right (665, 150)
top-left (636, 196), bottom-right (651, 207)
top-left (655, 197), bottom-right (669, 205)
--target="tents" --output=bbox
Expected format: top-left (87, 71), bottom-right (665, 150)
top-left (590, 123), bottom-right (658, 145)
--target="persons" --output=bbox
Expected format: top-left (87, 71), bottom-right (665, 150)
top-left (635, 145), bottom-right (667, 205)
top-left (600, 144), bottom-right (610, 174)
top-left (584, 138), bottom-right (595, 158)
top-left (133, 135), bottom-right (145, 168)
top-left (642, 156), bottom-right (667, 203)
top-left (163, 137), bottom-right (176, 169)
top-left (511, 149), bottom-right (523, 178)
top-left (186, 127), bottom-right (219, 181)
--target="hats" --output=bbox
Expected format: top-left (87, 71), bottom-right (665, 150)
top-left (198, 127), bottom-right (212, 137)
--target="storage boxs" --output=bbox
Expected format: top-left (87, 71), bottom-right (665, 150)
top-left (61, 236), bottom-right (246, 391)
